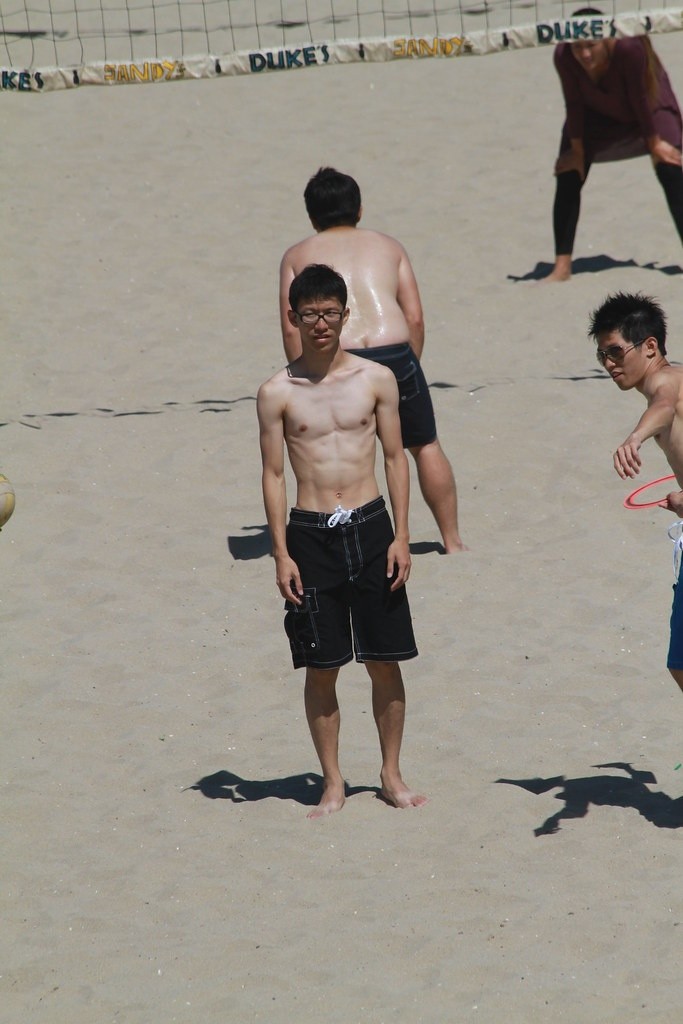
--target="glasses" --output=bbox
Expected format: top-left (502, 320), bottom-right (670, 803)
top-left (596, 339), bottom-right (646, 367)
top-left (293, 309), bottom-right (346, 325)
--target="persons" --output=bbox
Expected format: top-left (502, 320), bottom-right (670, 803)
top-left (539, 7), bottom-right (683, 284)
top-left (586, 288), bottom-right (683, 695)
top-left (257, 264), bottom-right (429, 819)
top-left (279, 166), bottom-right (474, 555)
